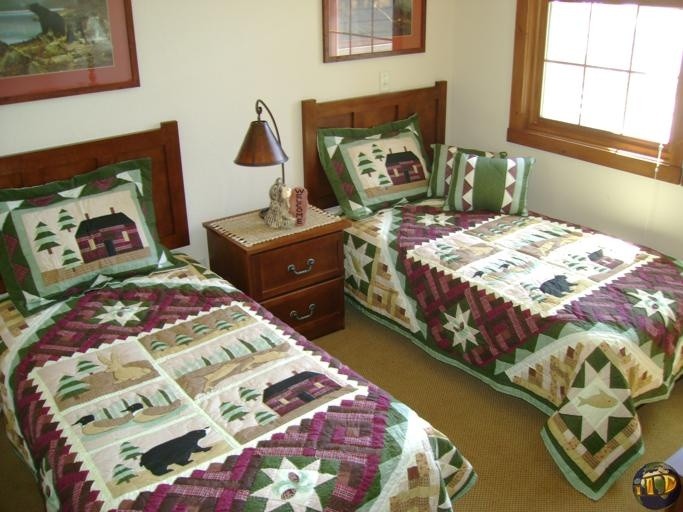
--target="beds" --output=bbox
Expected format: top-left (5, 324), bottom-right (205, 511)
top-left (302, 80), bottom-right (683, 501)
top-left (0, 121), bottom-right (477, 512)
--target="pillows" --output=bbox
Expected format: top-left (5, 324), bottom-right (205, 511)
top-left (427, 142), bottom-right (505, 203)
top-left (0, 157), bottom-right (185, 318)
top-left (317, 114), bottom-right (431, 220)
top-left (443, 151), bottom-right (535, 218)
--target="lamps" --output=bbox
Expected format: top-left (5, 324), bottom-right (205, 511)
top-left (232, 101), bottom-right (287, 220)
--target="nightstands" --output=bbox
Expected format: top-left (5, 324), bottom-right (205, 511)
top-left (203, 198), bottom-right (351, 341)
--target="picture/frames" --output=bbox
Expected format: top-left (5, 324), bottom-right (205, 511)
top-left (0, 0), bottom-right (142, 106)
top-left (321, 1), bottom-right (427, 64)
